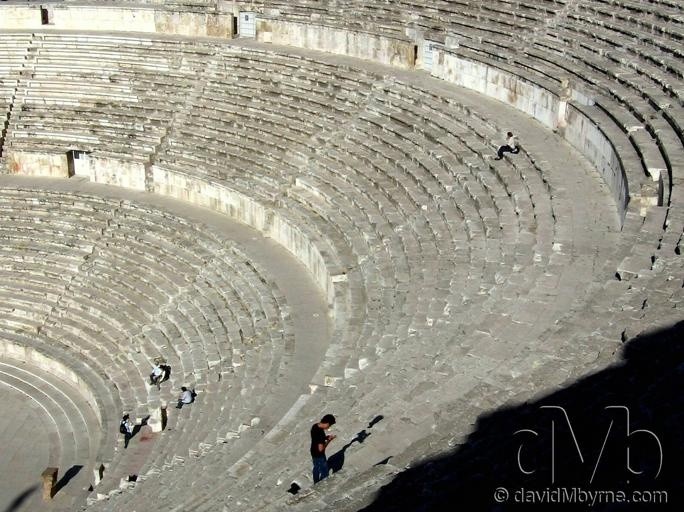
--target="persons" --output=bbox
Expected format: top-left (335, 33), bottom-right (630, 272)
top-left (494, 131), bottom-right (516, 160)
top-left (174, 386), bottom-right (193, 409)
top-left (309, 414), bottom-right (337, 485)
top-left (152, 365), bottom-right (167, 390)
top-left (119, 414), bottom-right (132, 448)
top-left (148, 361), bottom-right (160, 386)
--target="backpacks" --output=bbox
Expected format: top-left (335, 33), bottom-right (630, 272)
top-left (120, 421), bottom-right (127, 434)
